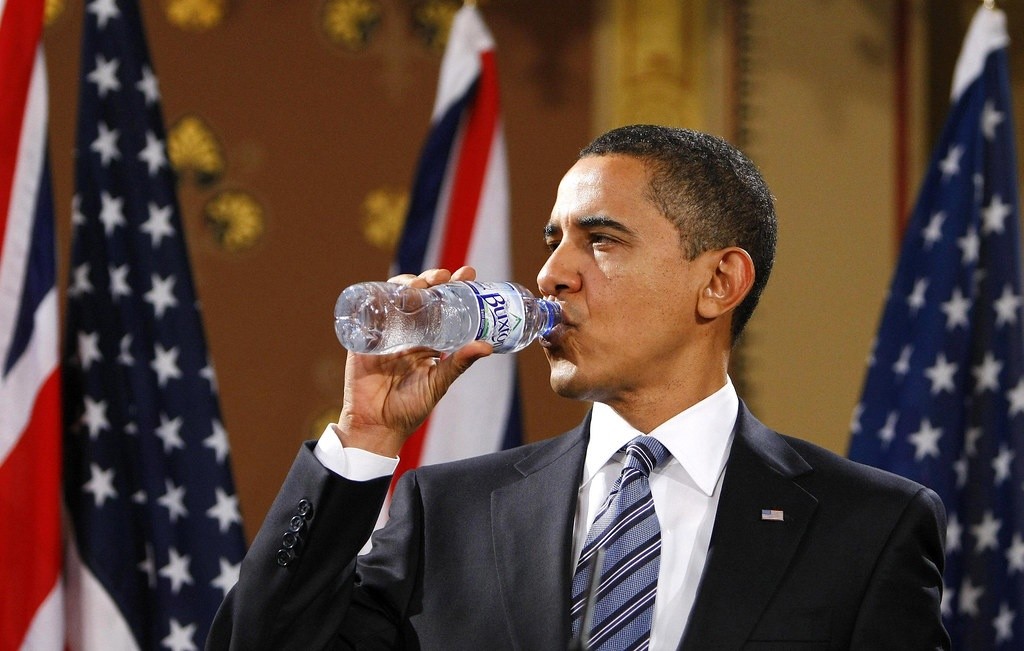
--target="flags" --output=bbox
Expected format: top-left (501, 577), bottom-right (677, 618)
top-left (0, 0), bottom-right (247, 651)
top-left (843, 0), bottom-right (1024, 651)
top-left (357, 5), bottom-right (524, 559)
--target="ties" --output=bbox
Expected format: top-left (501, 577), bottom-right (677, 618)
top-left (568, 436), bottom-right (671, 651)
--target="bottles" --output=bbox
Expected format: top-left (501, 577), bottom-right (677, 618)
top-left (335, 281), bottom-right (562, 356)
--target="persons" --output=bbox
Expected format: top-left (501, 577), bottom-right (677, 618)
top-left (204, 124), bottom-right (952, 651)
